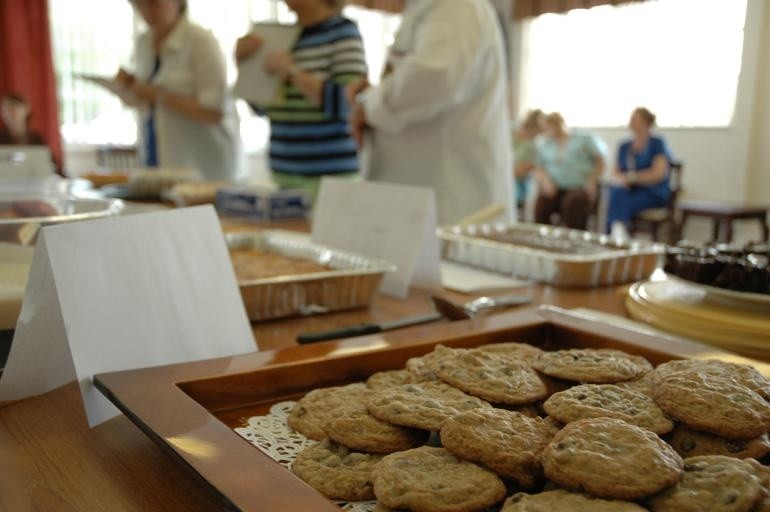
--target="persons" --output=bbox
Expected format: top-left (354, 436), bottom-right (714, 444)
top-left (0, 92), bottom-right (65, 184)
top-left (235, 0), bottom-right (369, 202)
top-left (532, 112), bottom-right (610, 229)
top-left (606, 106), bottom-right (673, 237)
top-left (511, 109), bottom-right (545, 215)
top-left (124, 0), bottom-right (244, 183)
top-left (346, 0), bottom-right (521, 228)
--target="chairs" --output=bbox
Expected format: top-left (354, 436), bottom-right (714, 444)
top-left (632, 161), bottom-right (682, 241)
top-left (552, 184), bottom-right (600, 231)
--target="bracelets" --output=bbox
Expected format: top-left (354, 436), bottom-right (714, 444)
top-left (287, 64), bottom-right (300, 78)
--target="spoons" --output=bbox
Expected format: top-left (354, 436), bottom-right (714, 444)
top-left (432, 293), bottom-right (530, 319)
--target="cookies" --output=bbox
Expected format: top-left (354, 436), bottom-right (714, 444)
top-left (287, 343), bottom-right (770, 511)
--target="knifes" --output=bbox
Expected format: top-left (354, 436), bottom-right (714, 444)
top-left (297, 312), bottom-right (441, 343)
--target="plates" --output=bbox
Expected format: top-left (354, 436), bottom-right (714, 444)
top-left (662, 272), bottom-right (770, 309)
top-left (1, 194), bottom-right (125, 226)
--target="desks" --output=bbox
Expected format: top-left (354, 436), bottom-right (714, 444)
top-left (677, 202), bottom-right (770, 247)
